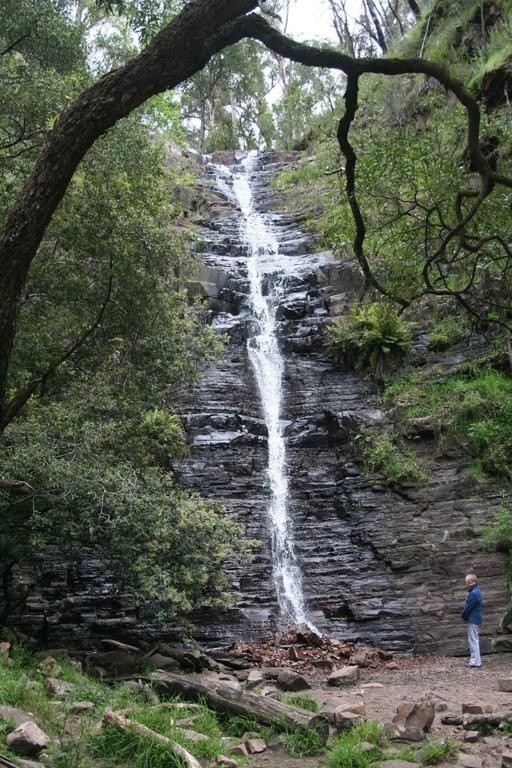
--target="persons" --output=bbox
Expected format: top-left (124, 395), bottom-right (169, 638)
top-left (461, 574), bottom-right (483, 668)
